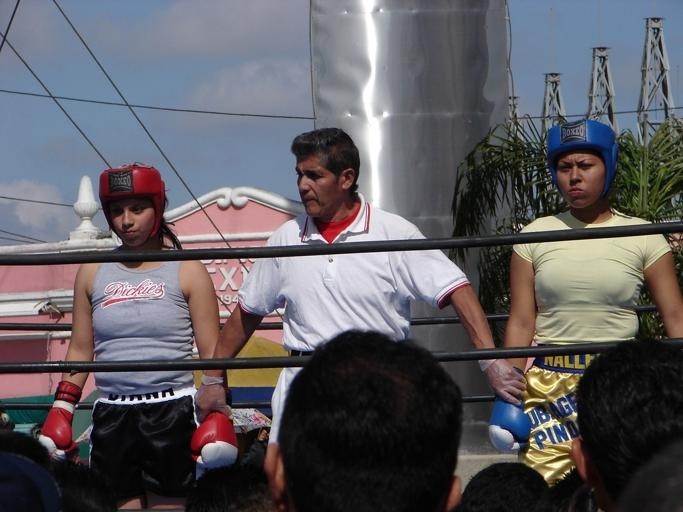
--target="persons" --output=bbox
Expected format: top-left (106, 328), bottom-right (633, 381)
top-left (33, 160), bottom-right (241, 511)
top-left (193, 124), bottom-right (528, 487)
top-left (188, 330), bottom-right (460, 512)
top-left (485, 118), bottom-right (683, 487)
top-left (462, 337), bottom-right (683, 510)
top-left (2, 422), bottom-right (111, 510)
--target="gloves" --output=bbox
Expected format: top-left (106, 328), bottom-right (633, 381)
top-left (188, 385), bottom-right (242, 472)
top-left (487, 365), bottom-right (532, 456)
top-left (35, 379), bottom-right (84, 464)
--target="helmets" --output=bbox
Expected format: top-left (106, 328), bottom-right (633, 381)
top-left (96, 163), bottom-right (166, 251)
top-left (545, 118), bottom-right (619, 203)
